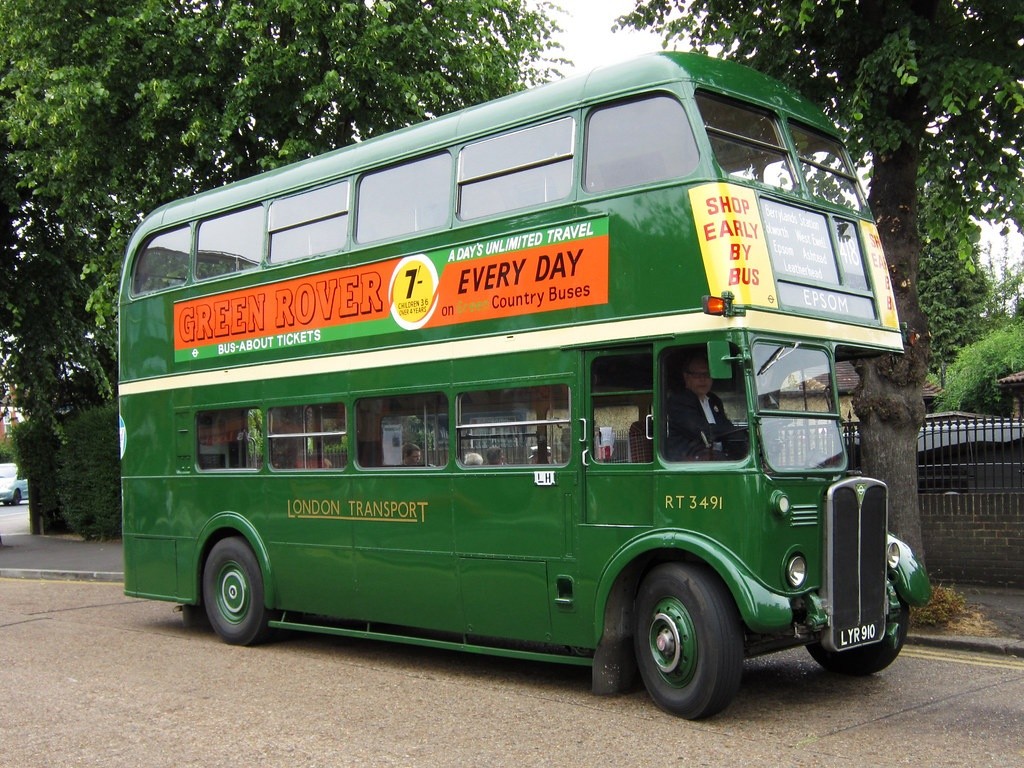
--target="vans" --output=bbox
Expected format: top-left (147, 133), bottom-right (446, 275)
top-left (811, 416), bottom-right (1024, 518)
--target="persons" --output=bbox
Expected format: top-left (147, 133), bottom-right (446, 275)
top-left (666, 350), bottom-right (746, 462)
top-left (399, 444), bottom-right (421, 465)
top-left (462, 444), bottom-right (552, 466)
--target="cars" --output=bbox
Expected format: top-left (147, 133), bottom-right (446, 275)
top-left (0, 462), bottom-right (29, 506)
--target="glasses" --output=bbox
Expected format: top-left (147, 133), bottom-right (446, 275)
top-left (684, 370), bottom-right (710, 378)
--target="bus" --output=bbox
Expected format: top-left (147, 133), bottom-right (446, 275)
top-left (119, 53), bottom-right (933, 724)
